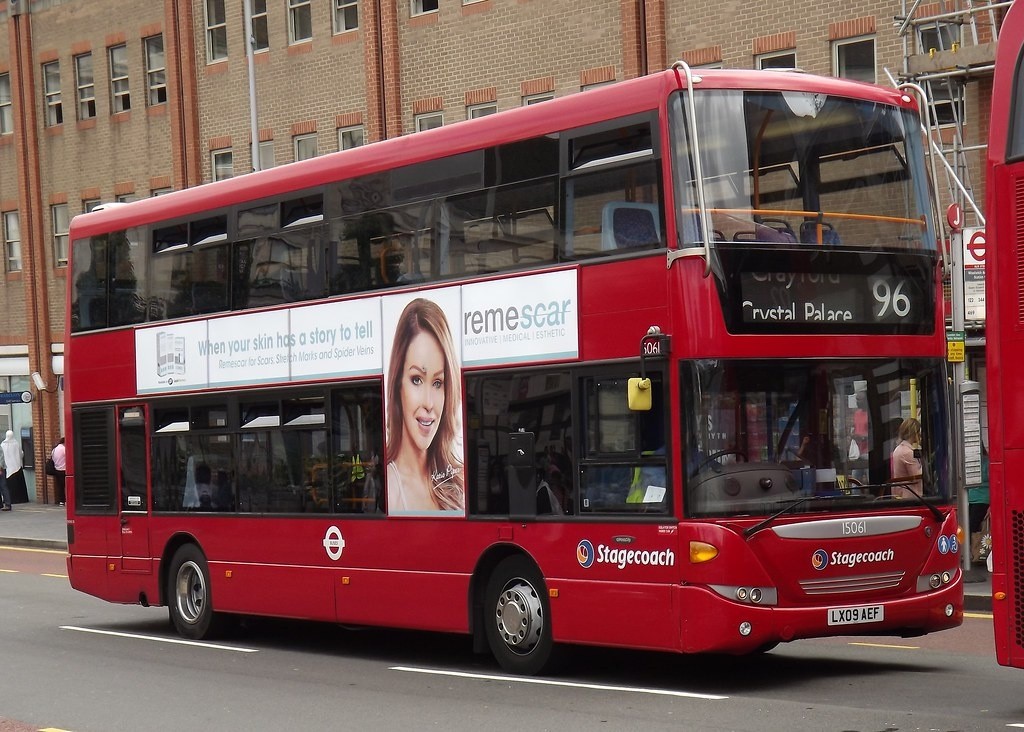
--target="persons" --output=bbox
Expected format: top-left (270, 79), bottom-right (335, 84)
top-left (0, 429), bottom-right (30, 511)
top-left (384, 298), bottom-right (463, 515)
top-left (843, 387), bottom-right (928, 501)
top-left (51, 436), bottom-right (68, 506)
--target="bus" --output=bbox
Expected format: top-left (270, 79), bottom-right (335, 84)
top-left (994, 0), bottom-right (1024, 671)
top-left (62, 59), bottom-right (963, 680)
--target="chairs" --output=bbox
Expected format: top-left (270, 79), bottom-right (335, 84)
top-left (69, 186), bottom-right (896, 528)
